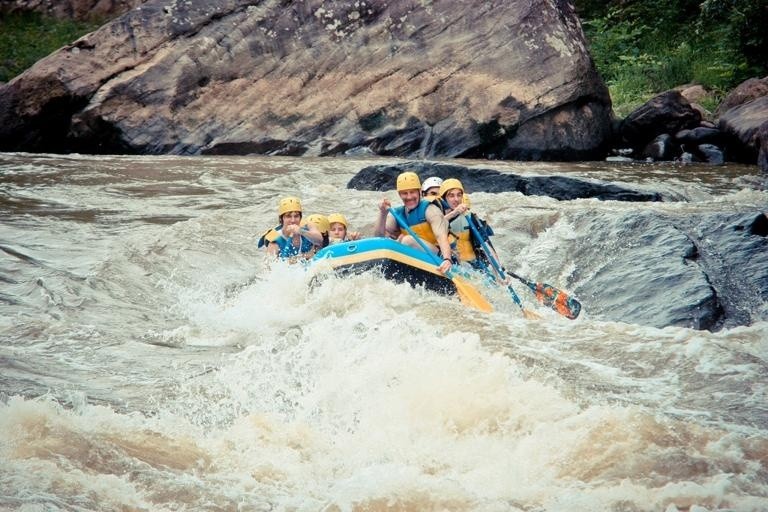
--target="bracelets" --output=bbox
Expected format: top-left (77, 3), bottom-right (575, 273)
top-left (444, 258), bottom-right (453, 265)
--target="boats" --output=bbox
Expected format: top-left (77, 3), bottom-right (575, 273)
top-left (308, 238), bottom-right (541, 318)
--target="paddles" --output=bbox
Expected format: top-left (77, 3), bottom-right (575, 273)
top-left (464, 209), bottom-right (544, 322)
top-left (480, 252), bottom-right (581, 319)
top-left (382, 201), bottom-right (494, 317)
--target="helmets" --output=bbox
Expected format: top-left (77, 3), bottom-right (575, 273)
top-left (278, 198), bottom-right (302, 217)
top-left (462, 195), bottom-right (470, 208)
top-left (329, 214), bottom-right (347, 229)
top-left (397, 172), bottom-right (422, 192)
top-left (301, 214), bottom-right (330, 233)
top-left (440, 179), bottom-right (463, 197)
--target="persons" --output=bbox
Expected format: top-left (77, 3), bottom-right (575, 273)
top-left (440, 177), bottom-right (512, 288)
top-left (327, 213), bottom-right (361, 243)
top-left (373, 170), bottom-right (454, 274)
top-left (260, 197), bottom-right (324, 265)
top-left (307, 214), bottom-right (343, 253)
